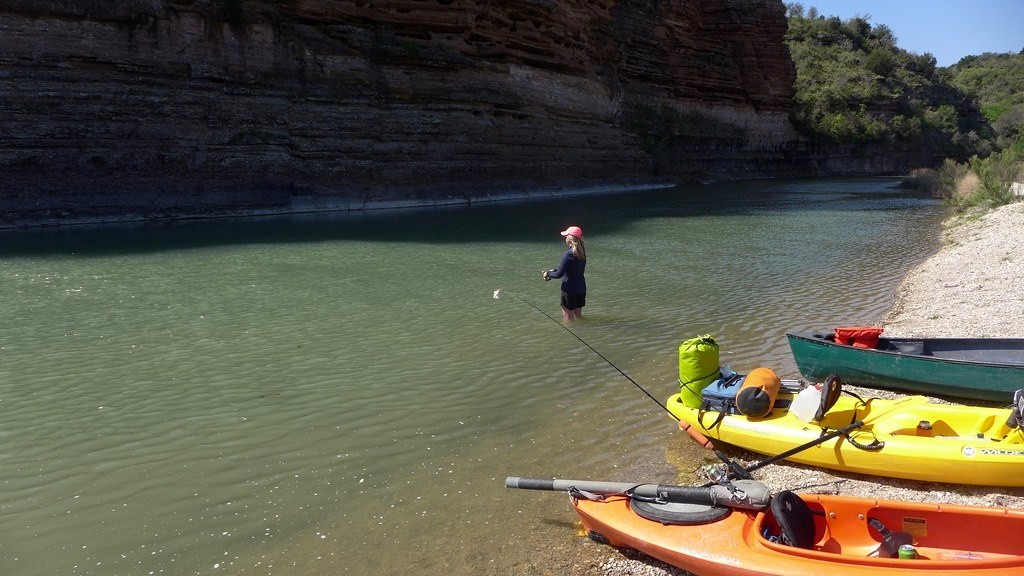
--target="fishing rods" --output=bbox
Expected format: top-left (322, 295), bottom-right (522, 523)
top-left (492, 287), bottom-right (755, 483)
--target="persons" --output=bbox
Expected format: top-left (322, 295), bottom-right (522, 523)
top-left (541, 226), bottom-right (587, 321)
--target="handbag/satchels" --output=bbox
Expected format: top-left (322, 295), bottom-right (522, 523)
top-left (697, 378), bottom-right (745, 430)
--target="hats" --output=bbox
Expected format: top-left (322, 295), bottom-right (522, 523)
top-left (561, 226), bottom-right (583, 238)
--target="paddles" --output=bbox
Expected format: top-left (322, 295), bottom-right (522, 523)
top-left (699, 395), bottom-right (928, 486)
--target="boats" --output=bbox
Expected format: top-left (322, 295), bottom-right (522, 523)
top-left (568, 488), bottom-right (1024, 576)
top-left (785, 332), bottom-right (1024, 402)
top-left (667, 376), bottom-right (1024, 488)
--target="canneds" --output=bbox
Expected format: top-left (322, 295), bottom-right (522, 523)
top-left (897, 544), bottom-right (916, 559)
